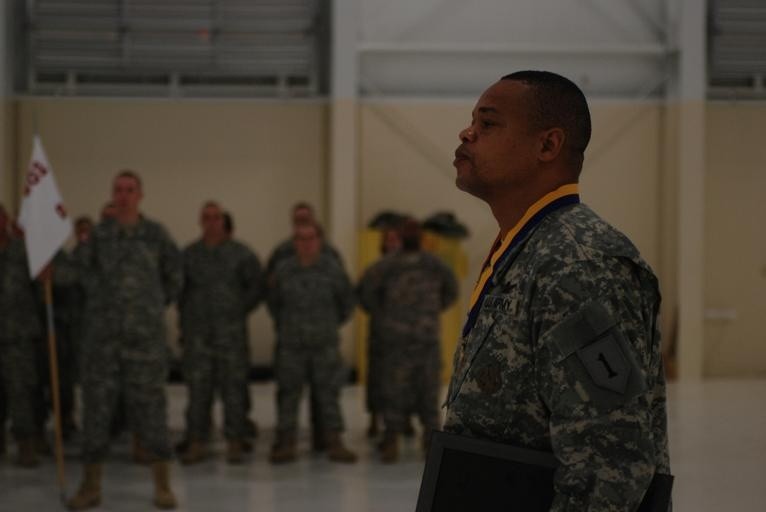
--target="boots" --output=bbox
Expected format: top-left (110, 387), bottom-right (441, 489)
top-left (3, 414), bottom-right (436, 468)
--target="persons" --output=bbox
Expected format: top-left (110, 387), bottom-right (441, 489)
top-left (175, 202), bottom-right (265, 465)
top-left (1, 170), bottom-right (184, 509)
top-left (442, 69), bottom-right (673, 511)
top-left (355, 219), bottom-right (461, 466)
top-left (262, 202), bottom-right (358, 465)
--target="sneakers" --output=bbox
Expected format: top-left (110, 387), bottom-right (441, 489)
top-left (152, 460), bottom-right (175, 507)
top-left (67, 462), bottom-right (103, 510)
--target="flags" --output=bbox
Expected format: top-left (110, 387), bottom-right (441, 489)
top-left (16, 137), bottom-right (74, 280)
